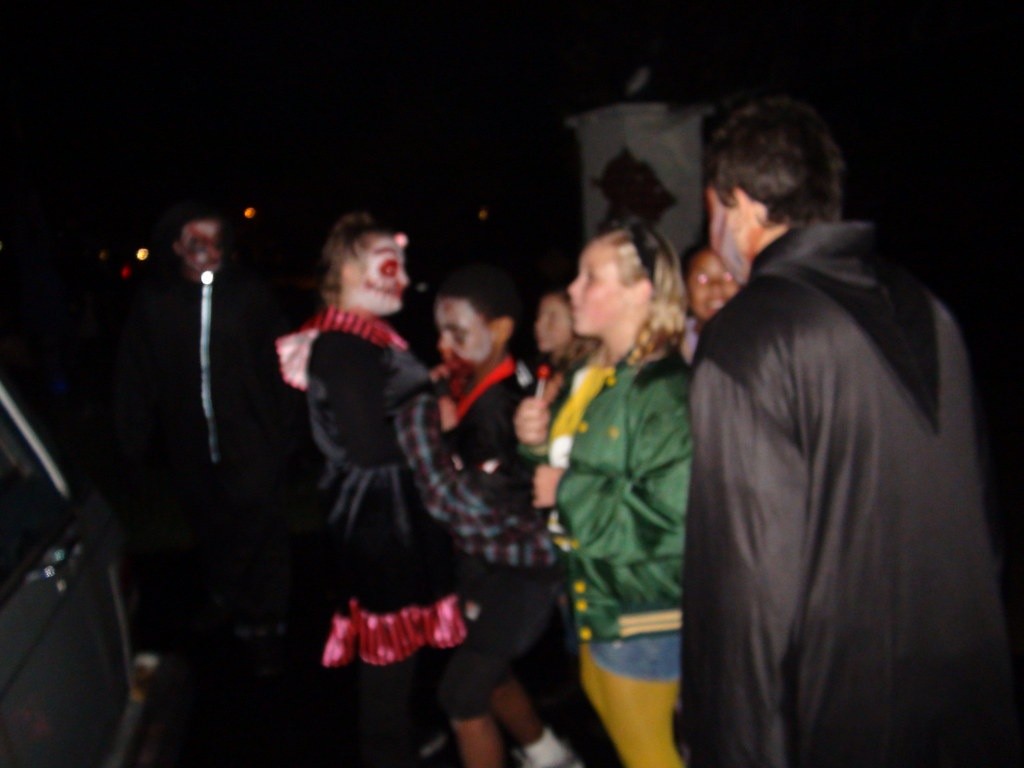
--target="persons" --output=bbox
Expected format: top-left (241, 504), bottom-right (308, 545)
top-left (512, 211), bottom-right (702, 768)
top-left (535, 288), bottom-right (573, 370)
top-left (274, 211), bottom-right (568, 768)
top-left (114, 206), bottom-right (302, 637)
top-left (682, 79), bottom-right (1024, 767)
top-left (418, 265), bottom-right (587, 766)
top-left (675, 241), bottom-right (741, 368)
